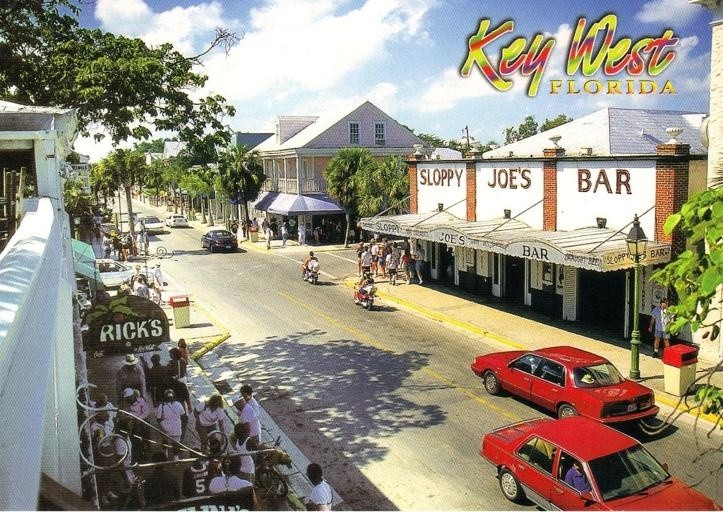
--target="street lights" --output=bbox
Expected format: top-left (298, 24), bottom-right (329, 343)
top-left (139, 235), bottom-right (153, 299)
top-left (623, 208), bottom-right (652, 384)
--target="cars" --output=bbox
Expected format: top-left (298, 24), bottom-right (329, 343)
top-left (94, 258), bottom-right (159, 289)
top-left (477, 414), bottom-right (722, 510)
top-left (298, 258), bottom-right (321, 285)
top-left (353, 280), bottom-right (375, 310)
top-left (164, 213), bottom-right (190, 229)
top-left (199, 229), bottom-right (240, 254)
top-left (469, 344), bottom-right (660, 429)
top-left (138, 216), bottom-right (167, 235)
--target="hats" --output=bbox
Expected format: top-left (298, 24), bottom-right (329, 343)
top-left (230, 395), bottom-right (244, 408)
top-left (122, 354), bottom-right (139, 366)
top-left (123, 387), bottom-right (134, 398)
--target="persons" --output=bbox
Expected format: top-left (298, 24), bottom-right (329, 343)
top-left (566, 458), bottom-right (593, 491)
top-left (355, 238), bottom-right (425, 305)
top-left (230, 216), bottom-right (322, 281)
top-left (90, 338), bottom-right (261, 511)
top-left (307, 463), bottom-right (332, 511)
top-left (648, 298), bottom-right (675, 358)
top-left (529, 357), bottom-right (549, 378)
top-left (100, 231), bottom-right (164, 301)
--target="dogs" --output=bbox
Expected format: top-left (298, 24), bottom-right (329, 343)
top-left (258, 445), bottom-right (293, 469)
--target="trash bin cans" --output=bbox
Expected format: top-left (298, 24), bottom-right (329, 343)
top-left (248, 227), bottom-right (258, 242)
top-left (662, 343), bottom-right (699, 397)
top-left (170, 295), bottom-right (190, 329)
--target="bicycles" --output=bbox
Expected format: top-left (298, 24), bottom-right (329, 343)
top-left (231, 431), bottom-right (294, 507)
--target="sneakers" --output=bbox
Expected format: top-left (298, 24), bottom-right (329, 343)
top-left (652, 351), bottom-right (659, 359)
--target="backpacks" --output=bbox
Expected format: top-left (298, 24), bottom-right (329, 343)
top-left (312, 264), bottom-right (319, 274)
